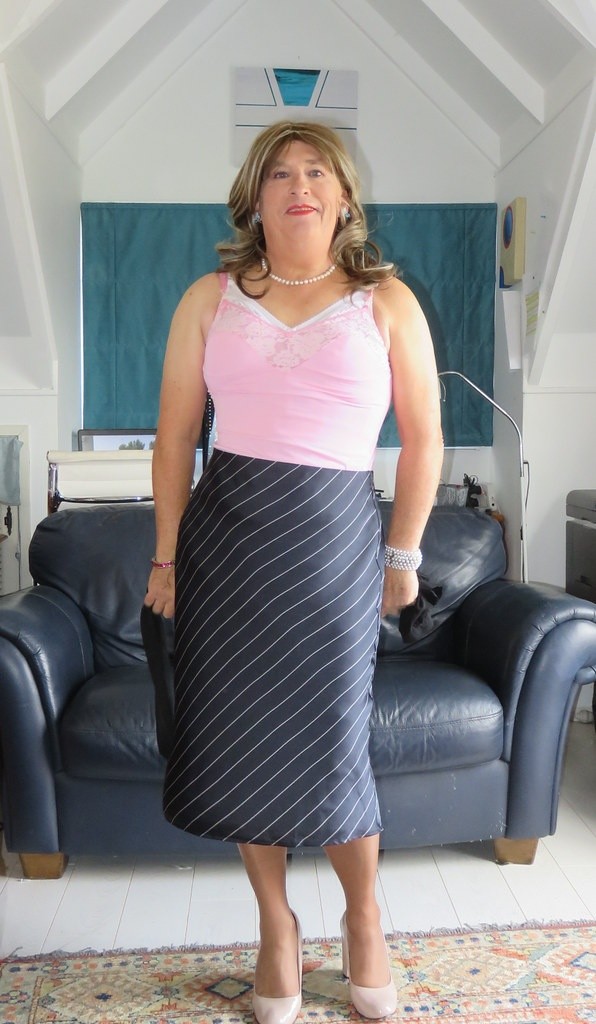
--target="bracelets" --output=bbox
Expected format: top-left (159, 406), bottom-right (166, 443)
top-left (385, 543), bottom-right (423, 570)
top-left (151, 555), bottom-right (175, 568)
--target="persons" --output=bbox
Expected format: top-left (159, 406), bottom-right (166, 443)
top-left (143, 120), bottom-right (446, 1023)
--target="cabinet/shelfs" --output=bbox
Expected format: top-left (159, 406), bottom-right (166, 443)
top-left (565, 487), bottom-right (596, 604)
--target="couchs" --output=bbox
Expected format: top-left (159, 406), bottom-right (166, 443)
top-left (1, 502), bottom-right (596, 881)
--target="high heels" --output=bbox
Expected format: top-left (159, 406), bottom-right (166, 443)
top-left (339, 910), bottom-right (397, 1019)
top-left (251, 908), bottom-right (304, 1024)
